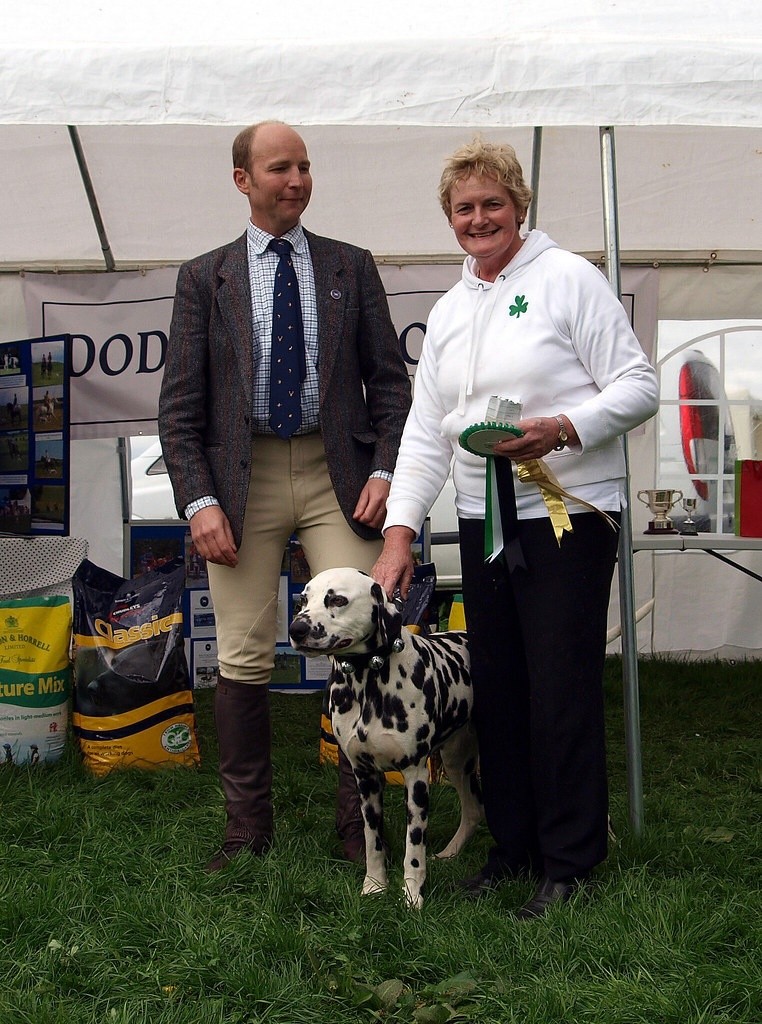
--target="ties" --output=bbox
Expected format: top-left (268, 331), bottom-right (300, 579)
top-left (268, 239), bottom-right (306, 440)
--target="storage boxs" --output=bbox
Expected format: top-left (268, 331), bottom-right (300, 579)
top-left (182, 573), bottom-right (333, 695)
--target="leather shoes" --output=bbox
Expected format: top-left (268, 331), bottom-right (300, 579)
top-left (514, 874), bottom-right (592, 919)
top-left (458, 870), bottom-right (534, 901)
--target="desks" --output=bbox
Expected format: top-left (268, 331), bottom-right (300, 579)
top-left (631, 532), bottom-right (762, 582)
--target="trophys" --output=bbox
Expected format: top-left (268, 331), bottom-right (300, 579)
top-left (677, 496), bottom-right (702, 536)
top-left (637, 489), bottom-right (683, 534)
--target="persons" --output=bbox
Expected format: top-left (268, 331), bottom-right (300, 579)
top-left (13, 394), bottom-right (18, 412)
top-left (371, 135), bottom-right (660, 922)
top-left (158, 121), bottom-right (412, 870)
top-left (45, 450), bottom-right (50, 463)
top-left (44, 391), bottom-right (51, 413)
top-left (42, 352), bottom-right (52, 363)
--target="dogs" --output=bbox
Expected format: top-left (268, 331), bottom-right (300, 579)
top-left (286, 564), bottom-right (625, 912)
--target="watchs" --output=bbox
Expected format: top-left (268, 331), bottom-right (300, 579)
top-left (552, 416), bottom-right (568, 451)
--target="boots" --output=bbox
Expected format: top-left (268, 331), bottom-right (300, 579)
top-left (205, 671), bottom-right (274, 873)
top-left (337, 751), bottom-right (366, 864)
